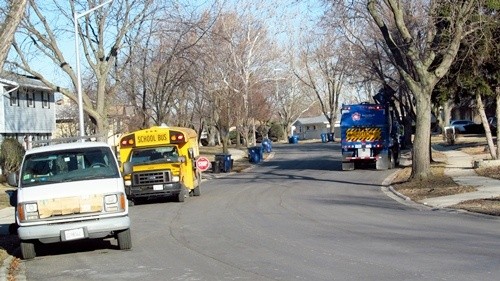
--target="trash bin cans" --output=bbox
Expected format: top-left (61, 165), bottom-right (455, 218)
top-left (321, 133), bottom-right (326, 142)
top-left (247, 147), bottom-right (263, 162)
top-left (231, 160), bottom-right (233, 169)
top-left (211, 161), bottom-right (220, 174)
top-left (291, 136), bottom-right (298, 144)
top-left (261, 140), bottom-right (272, 152)
top-left (327, 132), bottom-right (335, 141)
top-left (215, 154), bottom-right (232, 173)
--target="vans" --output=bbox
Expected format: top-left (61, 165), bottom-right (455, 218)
top-left (7, 141), bottom-right (132, 261)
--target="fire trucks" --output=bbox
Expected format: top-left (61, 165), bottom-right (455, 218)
top-left (117, 124), bottom-right (202, 203)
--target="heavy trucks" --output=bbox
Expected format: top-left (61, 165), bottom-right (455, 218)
top-left (340, 103), bottom-right (402, 171)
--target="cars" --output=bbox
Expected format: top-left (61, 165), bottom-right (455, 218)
top-left (488, 117), bottom-right (497, 129)
top-left (450, 119), bottom-right (476, 131)
